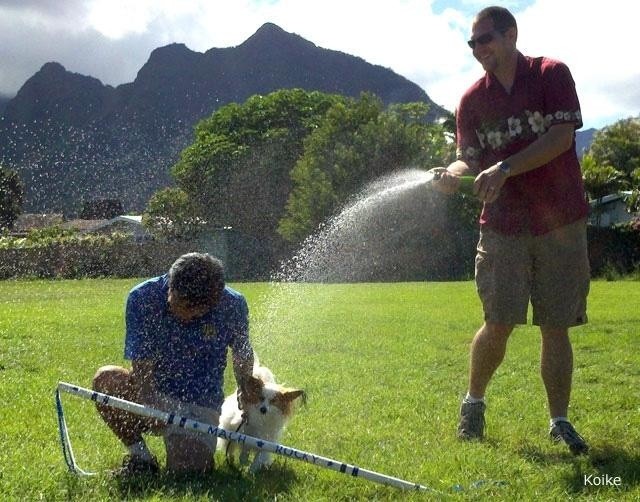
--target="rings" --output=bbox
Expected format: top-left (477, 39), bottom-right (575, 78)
top-left (489, 186), bottom-right (495, 191)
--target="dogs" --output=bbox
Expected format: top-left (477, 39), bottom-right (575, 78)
top-left (216, 350), bottom-right (309, 473)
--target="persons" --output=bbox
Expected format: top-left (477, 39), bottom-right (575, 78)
top-left (89, 252), bottom-right (268, 493)
top-left (426, 3), bottom-right (594, 459)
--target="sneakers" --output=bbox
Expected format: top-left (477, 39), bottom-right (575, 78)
top-left (112, 455), bottom-right (160, 480)
top-left (550, 421), bottom-right (587, 454)
top-left (454, 400), bottom-right (485, 441)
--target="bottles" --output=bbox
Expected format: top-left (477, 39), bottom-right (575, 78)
top-left (432, 173), bottom-right (485, 200)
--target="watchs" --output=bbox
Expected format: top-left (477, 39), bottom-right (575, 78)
top-left (497, 159), bottom-right (512, 179)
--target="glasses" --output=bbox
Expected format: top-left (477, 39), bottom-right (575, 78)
top-left (468, 28), bottom-right (506, 51)
